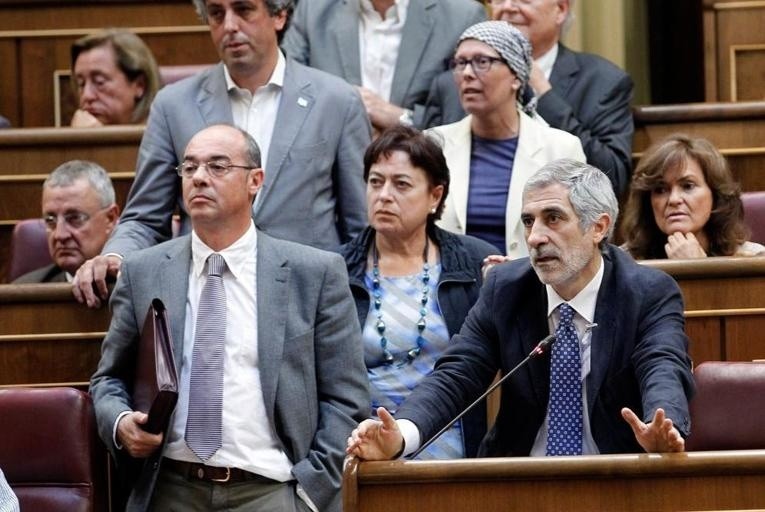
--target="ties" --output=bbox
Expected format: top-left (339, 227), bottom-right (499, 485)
top-left (546, 304), bottom-right (583, 456)
top-left (184, 254), bottom-right (225, 461)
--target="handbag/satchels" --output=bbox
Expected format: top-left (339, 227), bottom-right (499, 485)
top-left (133, 299), bottom-right (179, 432)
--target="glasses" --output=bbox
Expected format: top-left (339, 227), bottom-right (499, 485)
top-left (451, 56), bottom-right (504, 75)
top-left (43, 206), bottom-right (109, 230)
top-left (175, 161), bottom-right (254, 178)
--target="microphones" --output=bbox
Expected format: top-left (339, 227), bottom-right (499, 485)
top-left (404, 333), bottom-right (558, 459)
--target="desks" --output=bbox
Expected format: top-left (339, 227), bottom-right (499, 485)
top-left (0, 0), bottom-right (220, 510)
top-left (339, 2), bottom-right (764, 512)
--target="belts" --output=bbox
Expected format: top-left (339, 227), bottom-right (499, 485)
top-left (165, 459), bottom-right (248, 483)
